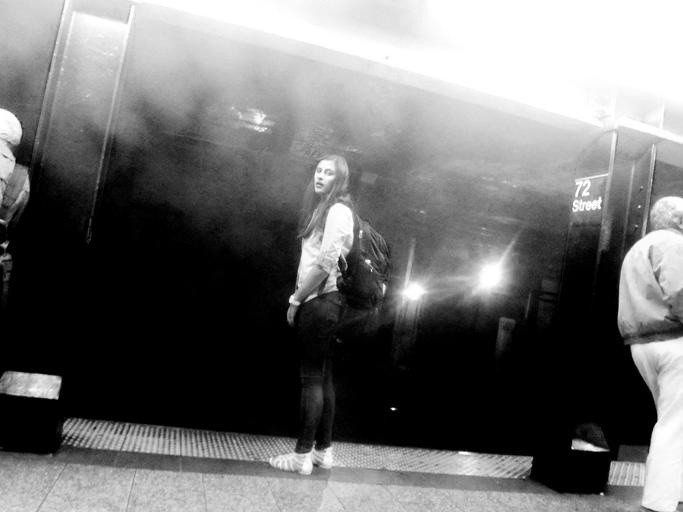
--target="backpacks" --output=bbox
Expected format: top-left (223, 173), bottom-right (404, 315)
top-left (321, 199), bottom-right (392, 311)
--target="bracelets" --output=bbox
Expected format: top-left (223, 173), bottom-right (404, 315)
top-left (288, 295), bottom-right (301, 307)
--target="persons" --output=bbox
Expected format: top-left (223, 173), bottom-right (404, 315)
top-left (1, 108), bottom-right (33, 294)
top-left (616, 194), bottom-right (683, 511)
top-left (268, 154), bottom-right (358, 476)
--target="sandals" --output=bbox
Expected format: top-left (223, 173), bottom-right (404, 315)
top-left (269, 446), bottom-right (334, 475)
